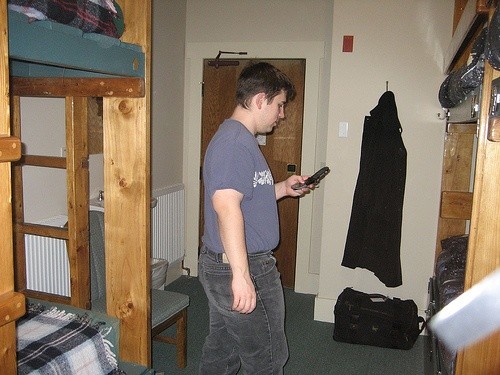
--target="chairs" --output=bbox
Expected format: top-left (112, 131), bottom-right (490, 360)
top-left (64, 212), bottom-right (189, 368)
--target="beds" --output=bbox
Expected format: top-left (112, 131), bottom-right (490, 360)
top-left (430, 0), bottom-right (500, 375)
top-left (0, 0), bottom-right (153, 375)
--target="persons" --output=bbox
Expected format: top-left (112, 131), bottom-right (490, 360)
top-left (197, 58), bottom-right (319, 375)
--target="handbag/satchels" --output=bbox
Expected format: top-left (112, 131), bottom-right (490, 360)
top-left (332, 286), bottom-right (426, 350)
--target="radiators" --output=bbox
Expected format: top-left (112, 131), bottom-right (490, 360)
top-left (23, 184), bottom-right (185, 298)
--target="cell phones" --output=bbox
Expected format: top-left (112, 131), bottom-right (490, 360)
top-left (291, 167), bottom-right (330, 190)
